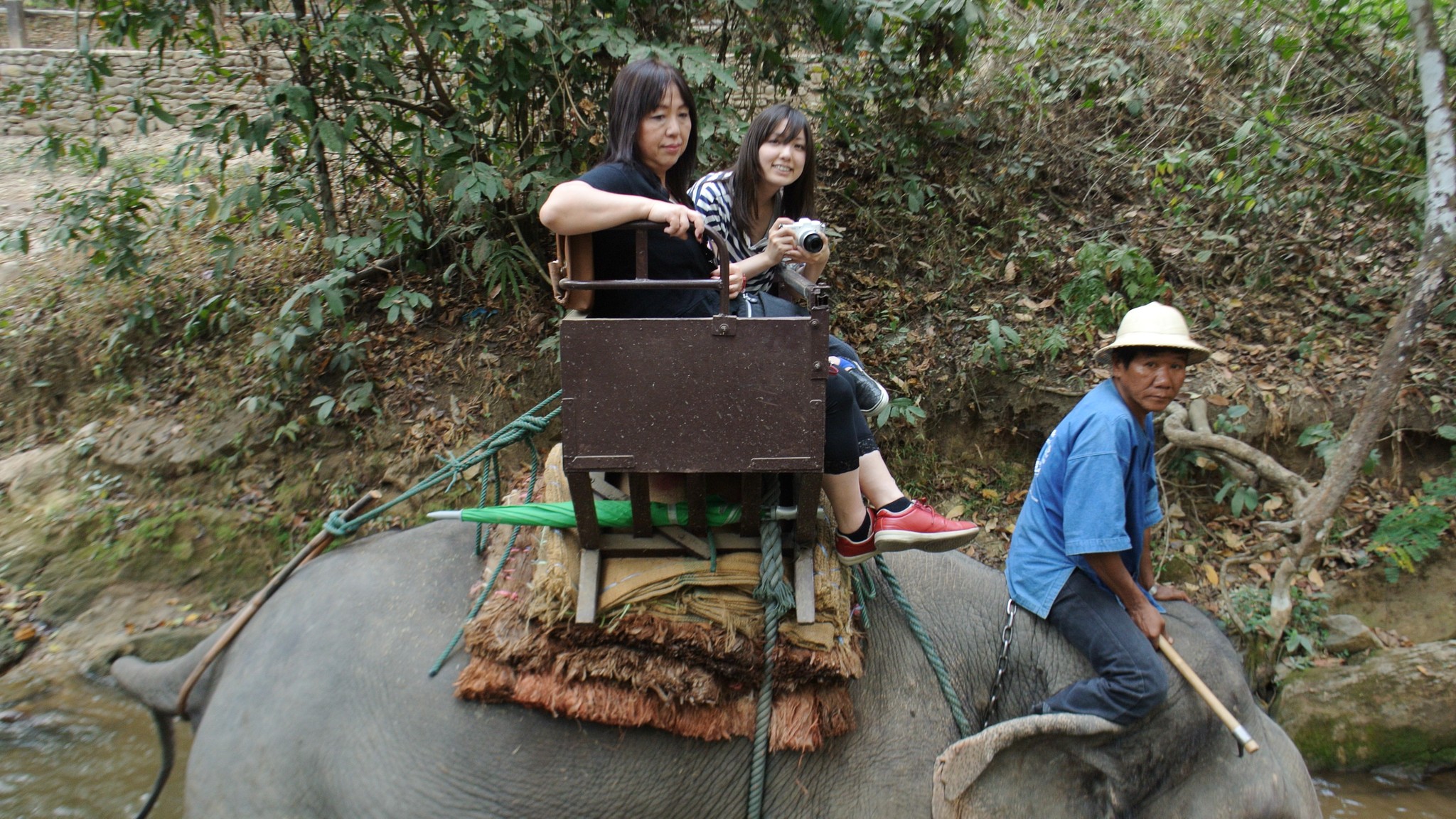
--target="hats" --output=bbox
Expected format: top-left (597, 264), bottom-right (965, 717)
top-left (1092, 299), bottom-right (1213, 367)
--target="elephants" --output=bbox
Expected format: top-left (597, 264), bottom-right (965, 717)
top-left (109, 518), bottom-right (1320, 819)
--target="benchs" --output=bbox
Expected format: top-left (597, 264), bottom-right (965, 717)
top-left (560, 221), bottom-right (830, 624)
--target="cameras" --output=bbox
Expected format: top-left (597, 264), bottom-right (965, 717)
top-left (781, 217), bottom-right (826, 253)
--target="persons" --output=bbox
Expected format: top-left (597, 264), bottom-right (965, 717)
top-left (685, 106), bottom-right (890, 416)
top-left (1000, 297), bottom-right (1211, 736)
top-left (537, 61), bottom-right (979, 565)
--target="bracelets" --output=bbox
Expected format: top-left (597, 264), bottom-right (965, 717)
top-left (741, 273), bottom-right (746, 292)
top-left (1148, 583), bottom-right (1158, 597)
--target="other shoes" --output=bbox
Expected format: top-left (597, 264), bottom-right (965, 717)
top-left (874, 496), bottom-right (982, 554)
top-left (834, 506), bottom-right (883, 566)
